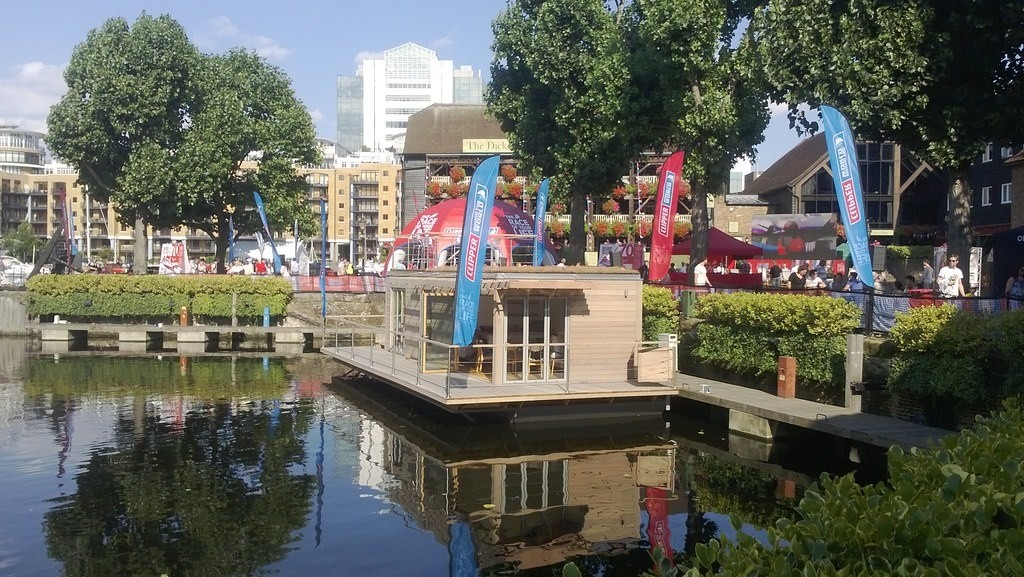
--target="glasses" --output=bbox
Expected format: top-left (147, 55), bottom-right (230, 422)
top-left (1020, 269), bottom-right (1024, 272)
top-left (949, 260), bottom-right (957, 262)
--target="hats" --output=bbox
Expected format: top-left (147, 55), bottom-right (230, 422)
top-left (872, 272), bottom-right (879, 280)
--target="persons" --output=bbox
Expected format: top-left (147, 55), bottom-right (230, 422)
top-left (920, 258), bottom-right (934, 288)
top-left (557, 258), bottom-right (566, 266)
top-left (189, 258), bottom-right (275, 275)
top-left (869, 239), bottom-right (881, 247)
top-left (337, 256), bottom-right (354, 275)
top-left (786, 266), bottom-right (807, 290)
top-left (694, 257), bottom-right (713, 287)
top-left (291, 257), bottom-right (299, 274)
top-left (89, 259), bottom-right (98, 271)
top-left (804, 269), bottom-right (827, 290)
top-left (936, 254), bottom-right (965, 298)
top-left (669, 256), bottom-right (1024, 298)
top-left (604, 238), bottom-right (628, 245)
top-left (639, 261), bottom-right (648, 278)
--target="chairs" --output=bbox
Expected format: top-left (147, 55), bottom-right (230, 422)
top-left (475, 342), bottom-right (564, 376)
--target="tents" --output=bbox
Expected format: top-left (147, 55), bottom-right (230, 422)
top-left (382, 197), bottom-right (567, 269)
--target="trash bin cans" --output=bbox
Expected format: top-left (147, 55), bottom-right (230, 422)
top-left (180, 306), bottom-right (193, 326)
top-left (309, 263), bottom-right (321, 276)
top-left (658, 334), bottom-right (678, 371)
top-left (180, 356), bottom-right (192, 377)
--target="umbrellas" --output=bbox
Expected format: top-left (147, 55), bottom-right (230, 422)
top-left (670, 225), bottom-right (764, 269)
